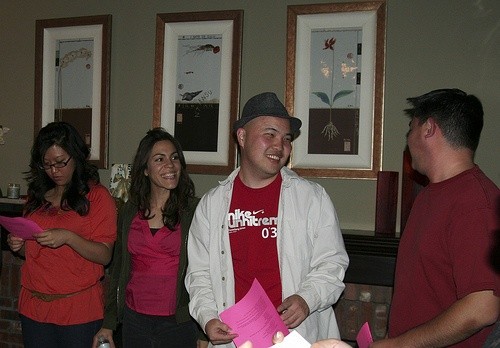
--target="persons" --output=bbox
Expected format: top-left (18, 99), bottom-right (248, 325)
top-left (184, 88), bottom-right (500, 348)
top-left (93, 126), bottom-right (209, 348)
top-left (6, 121), bottom-right (118, 348)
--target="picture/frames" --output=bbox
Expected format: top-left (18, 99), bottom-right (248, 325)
top-left (34, 14), bottom-right (113, 170)
top-left (151, 9), bottom-right (244, 176)
top-left (285, 1), bottom-right (387, 181)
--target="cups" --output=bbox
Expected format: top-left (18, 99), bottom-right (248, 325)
top-left (7, 184), bottom-right (20, 199)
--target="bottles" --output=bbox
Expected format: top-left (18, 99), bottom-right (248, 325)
top-left (96, 337), bottom-right (110, 348)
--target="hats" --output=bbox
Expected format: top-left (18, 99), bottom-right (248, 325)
top-left (232, 91), bottom-right (302, 141)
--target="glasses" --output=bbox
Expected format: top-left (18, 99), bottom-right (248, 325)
top-left (40, 157), bottom-right (72, 169)
